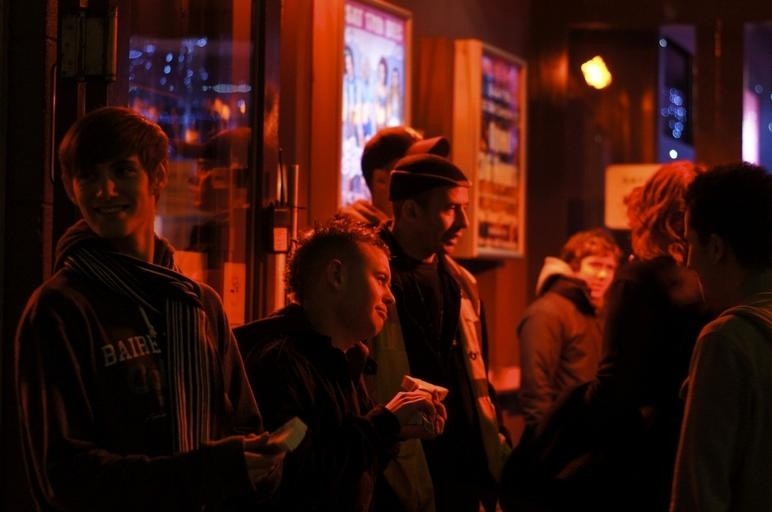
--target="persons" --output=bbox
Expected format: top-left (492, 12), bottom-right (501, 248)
top-left (487, 160), bottom-right (723, 511)
top-left (228, 220), bottom-right (447, 512)
top-left (169, 126), bottom-right (278, 267)
top-left (336, 125), bottom-right (453, 231)
top-left (356, 154), bottom-right (514, 511)
top-left (668, 160), bottom-right (772, 512)
top-left (13, 104), bottom-right (286, 512)
top-left (515, 228), bottom-right (623, 424)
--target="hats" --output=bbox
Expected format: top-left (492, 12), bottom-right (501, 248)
top-left (362, 125), bottom-right (452, 172)
